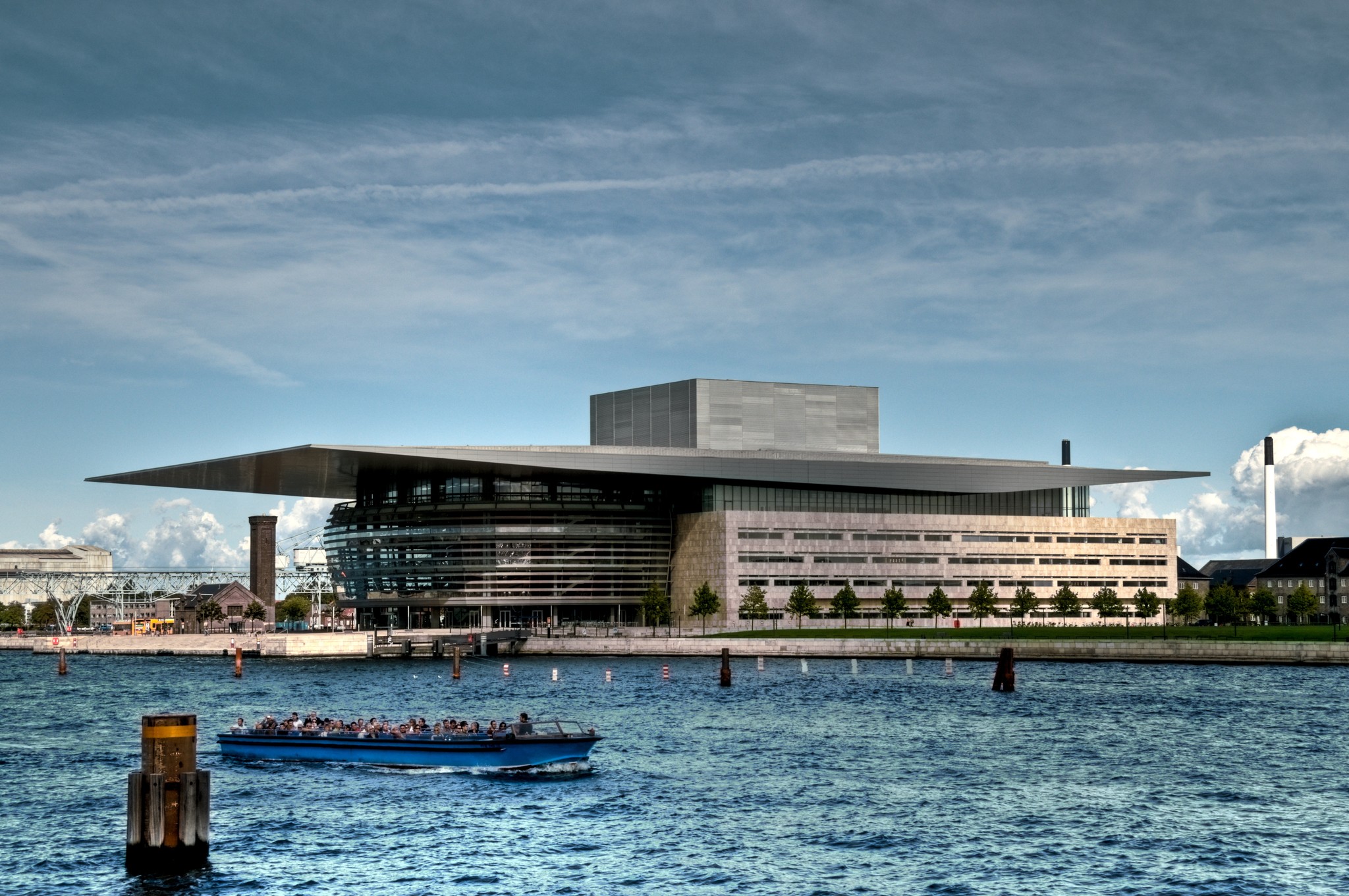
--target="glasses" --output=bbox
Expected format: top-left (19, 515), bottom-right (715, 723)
top-left (370, 728), bottom-right (374, 730)
top-left (312, 722), bottom-right (317, 723)
top-left (344, 728), bottom-right (347, 729)
top-left (410, 721), bottom-right (413, 723)
top-left (501, 726), bottom-right (506, 727)
top-left (307, 726), bottom-right (311, 727)
top-left (434, 730), bottom-right (438, 731)
top-left (416, 728), bottom-right (421, 729)
top-left (406, 727), bottom-right (408, 728)
top-left (331, 723), bottom-right (334, 724)
top-left (453, 725), bottom-right (456, 727)
top-left (457, 728), bottom-right (462, 729)
top-left (258, 723), bottom-right (262, 725)
top-left (419, 721), bottom-right (422, 723)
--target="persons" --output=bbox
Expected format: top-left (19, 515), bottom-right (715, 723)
top-left (909, 617), bottom-right (914, 628)
top-left (249, 711), bottom-right (506, 740)
top-left (387, 635), bottom-right (393, 646)
top-left (516, 713), bottom-right (533, 736)
top-left (906, 617), bottom-right (910, 627)
top-left (204, 624), bottom-right (210, 636)
top-left (230, 718), bottom-right (249, 735)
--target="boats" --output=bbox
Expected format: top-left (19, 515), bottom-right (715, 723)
top-left (217, 722), bottom-right (609, 777)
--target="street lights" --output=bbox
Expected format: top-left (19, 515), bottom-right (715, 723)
top-left (679, 609), bottom-right (680, 637)
top-left (773, 607), bottom-right (774, 639)
top-left (886, 606), bottom-right (888, 638)
top-left (1127, 606), bottom-right (1129, 639)
top-left (1163, 603), bottom-right (1165, 641)
top-left (1010, 604), bottom-right (1014, 639)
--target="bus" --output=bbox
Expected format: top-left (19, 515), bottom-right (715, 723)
top-left (112, 619), bottom-right (146, 635)
top-left (145, 619), bottom-right (175, 635)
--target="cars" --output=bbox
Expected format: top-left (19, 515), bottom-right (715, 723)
top-left (71, 625), bottom-right (111, 631)
top-left (1194, 619), bottom-right (1212, 627)
top-left (1264, 620), bottom-right (1268, 626)
top-left (44, 627), bottom-right (57, 632)
